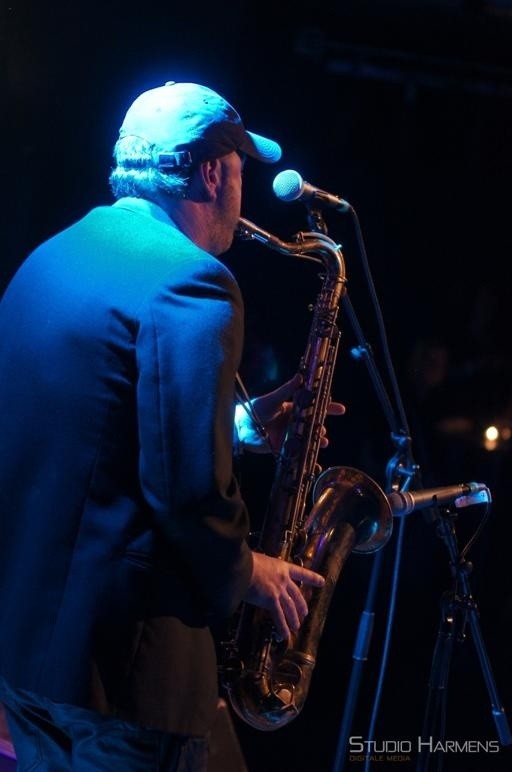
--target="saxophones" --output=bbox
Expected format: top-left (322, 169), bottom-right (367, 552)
top-left (218, 218), bottom-right (393, 731)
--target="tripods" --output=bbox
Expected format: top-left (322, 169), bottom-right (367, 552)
top-left (311, 211), bottom-right (512, 772)
top-left (415, 513), bottom-right (512, 772)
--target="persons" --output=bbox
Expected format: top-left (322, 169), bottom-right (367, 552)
top-left (1, 76), bottom-right (350, 769)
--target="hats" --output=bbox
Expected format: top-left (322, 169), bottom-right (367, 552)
top-left (116, 81), bottom-right (282, 169)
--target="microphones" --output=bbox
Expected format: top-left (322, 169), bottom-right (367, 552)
top-left (272, 169), bottom-right (354, 218)
top-left (387, 482), bottom-right (480, 516)
